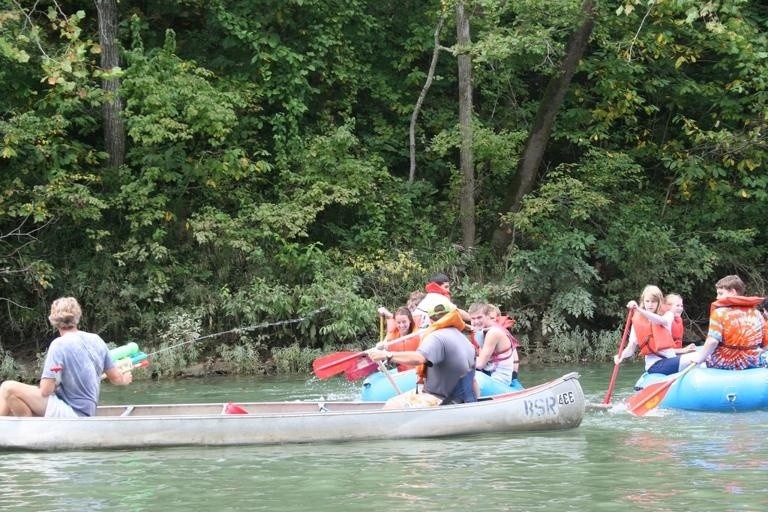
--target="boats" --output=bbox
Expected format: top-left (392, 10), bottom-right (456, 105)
top-left (360, 365), bottom-right (525, 402)
top-left (1, 372), bottom-right (586, 451)
top-left (634, 364), bottom-right (767, 411)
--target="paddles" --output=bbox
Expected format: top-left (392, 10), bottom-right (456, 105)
top-left (312, 327), bottom-right (429, 379)
top-left (344, 355), bottom-right (387, 383)
top-left (624, 357), bottom-right (707, 416)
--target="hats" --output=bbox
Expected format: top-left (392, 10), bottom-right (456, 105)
top-left (432, 273), bottom-right (450, 283)
top-left (427, 300), bottom-right (450, 317)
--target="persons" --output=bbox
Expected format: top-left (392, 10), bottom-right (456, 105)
top-left (613, 286), bottom-right (707, 375)
top-left (0, 296), bottom-right (134, 417)
top-left (367, 273), bottom-right (519, 411)
top-left (690, 274), bottom-right (768, 370)
top-left (662, 292), bottom-right (696, 356)
